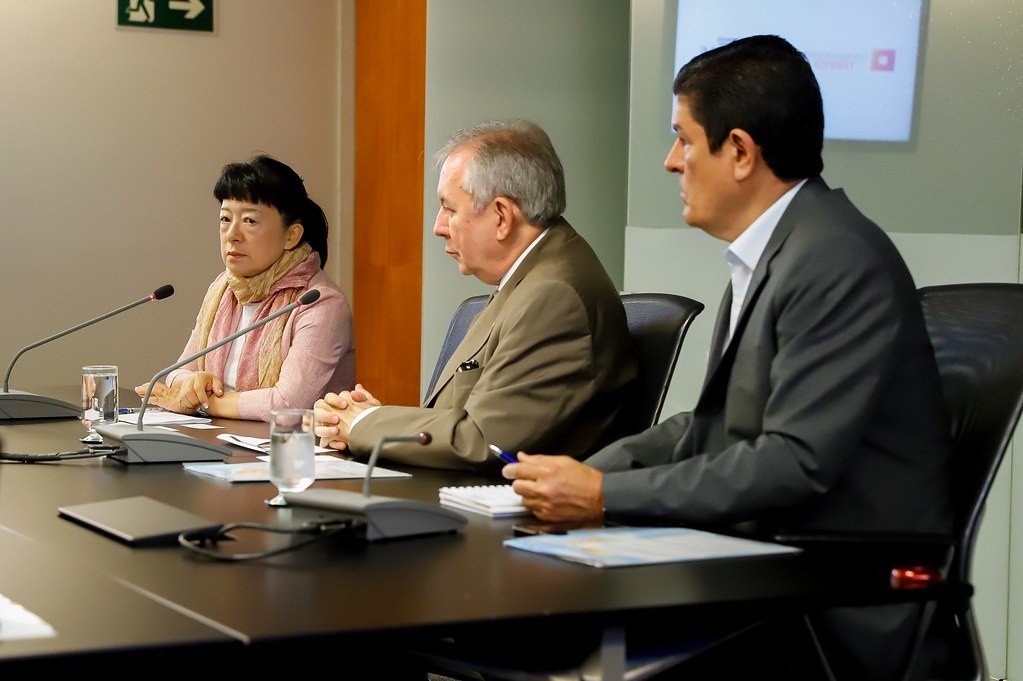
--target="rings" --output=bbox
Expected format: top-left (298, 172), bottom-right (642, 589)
top-left (177, 395), bottom-right (183, 402)
top-left (159, 387), bottom-right (164, 397)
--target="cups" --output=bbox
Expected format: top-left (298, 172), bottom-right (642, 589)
top-left (268, 409), bottom-right (317, 494)
top-left (80, 365), bottom-right (121, 443)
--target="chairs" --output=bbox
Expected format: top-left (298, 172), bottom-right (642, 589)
top-left (566, 290), bottom-right (705, 508)
top-left (734, 281), bottom-right (1023, 679)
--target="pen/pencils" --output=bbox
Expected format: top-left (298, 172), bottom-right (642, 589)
top-left (488, 442), bottom-right (519, 464)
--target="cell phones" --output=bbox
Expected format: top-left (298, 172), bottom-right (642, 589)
top-left (512, 519), bottom-right (608, 537)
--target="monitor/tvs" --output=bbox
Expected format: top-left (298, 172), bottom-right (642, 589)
top-left (663, 1), bottom-right (932, 151)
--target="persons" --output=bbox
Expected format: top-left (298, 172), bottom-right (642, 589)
top-left (134, 154), bottom-right (355, 422)
top-left (502, 32), bottom-right (944, 680)
top-left (301, 118), bottom-right (642, 477)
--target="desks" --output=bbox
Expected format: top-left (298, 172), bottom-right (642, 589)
top-left (0, 383), bottom-right (976, 681)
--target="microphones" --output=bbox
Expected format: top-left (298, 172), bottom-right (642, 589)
top-left (3, 284), bottom-right (176, 393)
top-left (137, 289), bottom-right (321, 429)
top-left (364, 431), bottom-right (432, 499)
